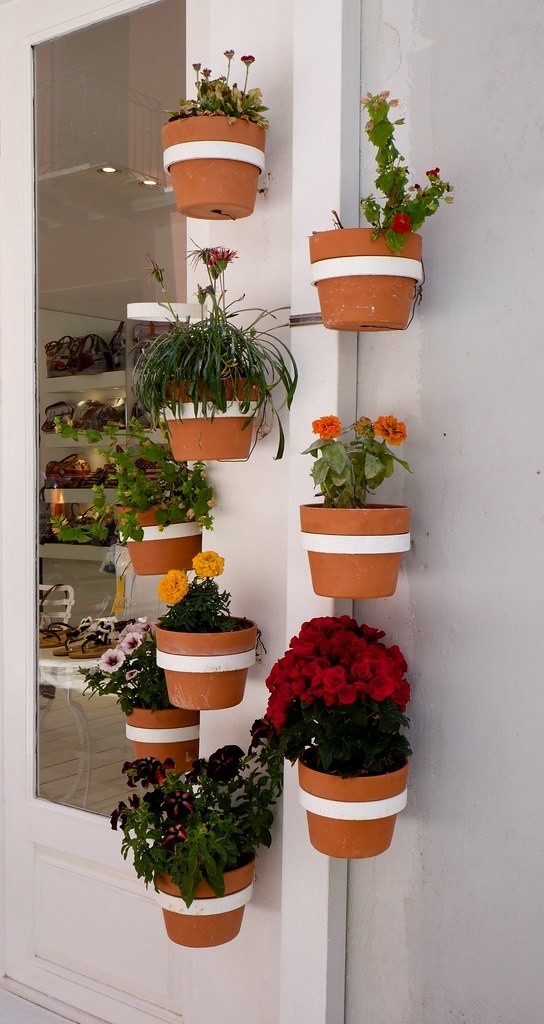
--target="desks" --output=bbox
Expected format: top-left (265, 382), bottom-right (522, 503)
top-left (38, 646), bottom-right (138, 810)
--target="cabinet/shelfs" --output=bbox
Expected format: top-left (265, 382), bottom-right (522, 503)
top-left (36, 369), bottom-right (181, 560)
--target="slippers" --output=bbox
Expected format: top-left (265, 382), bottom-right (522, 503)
top-left (40, 616), bottom-right (136, 659)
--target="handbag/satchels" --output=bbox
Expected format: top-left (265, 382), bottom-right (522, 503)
top-left (40, 612), bottom-right (52, 630)
top-left (39, 320), bottom-right (159, 546)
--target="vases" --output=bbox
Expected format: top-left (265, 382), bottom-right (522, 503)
top-left (161, 375), bottom-right (258, 460)
top-left (115, 501), bottom-right (203, 576)
top-left (159, 115), bottom-right (267, 220)
top-left (299, 746), bottom-right (410, 860)
top-left (308, 228), bottom-right (423, 333)
top-left (154, 617), bottom-right (257, 710)
top-left (125, 709), bottom-right (200, 774)
top-left (299, 504), bottom-right (411, 599)
top-left (152, 847), bottom-right (256, 950)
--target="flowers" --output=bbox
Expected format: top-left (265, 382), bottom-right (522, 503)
top-left (109, 719), bottom-right (283, 909)
top-left (155, 552), bottom-right (244, 634)
top-left (263, 615), bottom-right (414, 778)
top-left (47, 416), bottom-right (217, 545)
top-left (124, 235), bottom-right (299, 464)
top-left (159, 49), bottom-right (271, 130)
top-left (357, 89), bottom-right (456, 254)
top-left (300, 415), bottom-right (412, 508)
top-left (71, 615), bottom-right (175, 716)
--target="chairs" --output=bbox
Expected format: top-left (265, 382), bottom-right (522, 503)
top-left (37, 584), bottom-right (91, 811)
